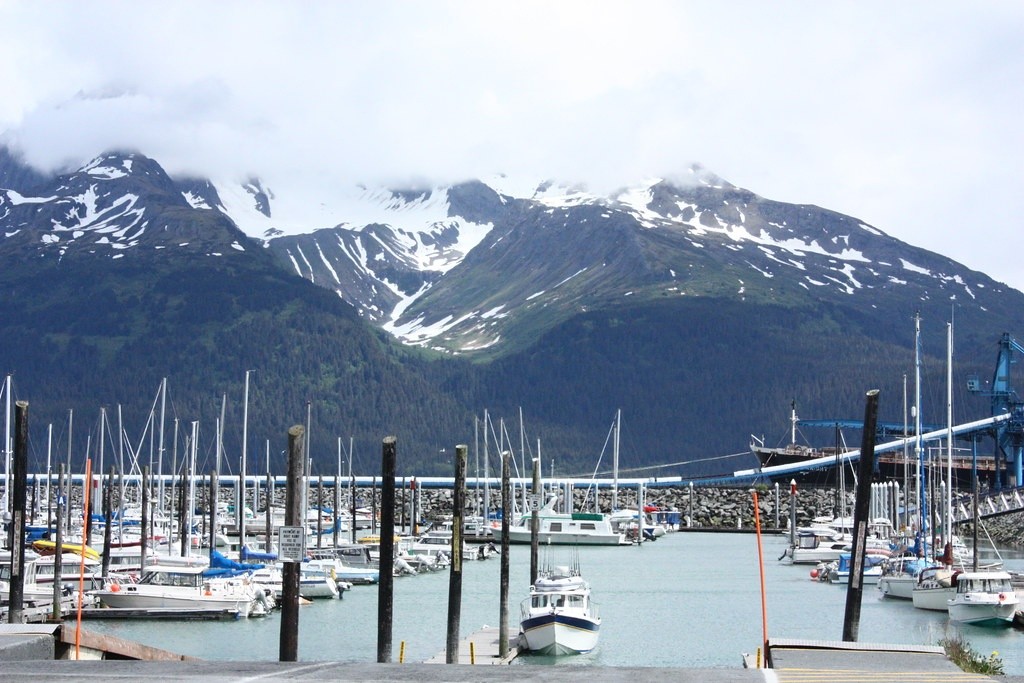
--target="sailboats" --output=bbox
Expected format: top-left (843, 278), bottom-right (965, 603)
top-left (777, 308), bottom-right (1024, 629)
top-left (0, 373), bottom-right (680, 625)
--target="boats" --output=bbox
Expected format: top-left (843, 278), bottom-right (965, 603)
top-left (517, 530), bottom-right (603, 658)
